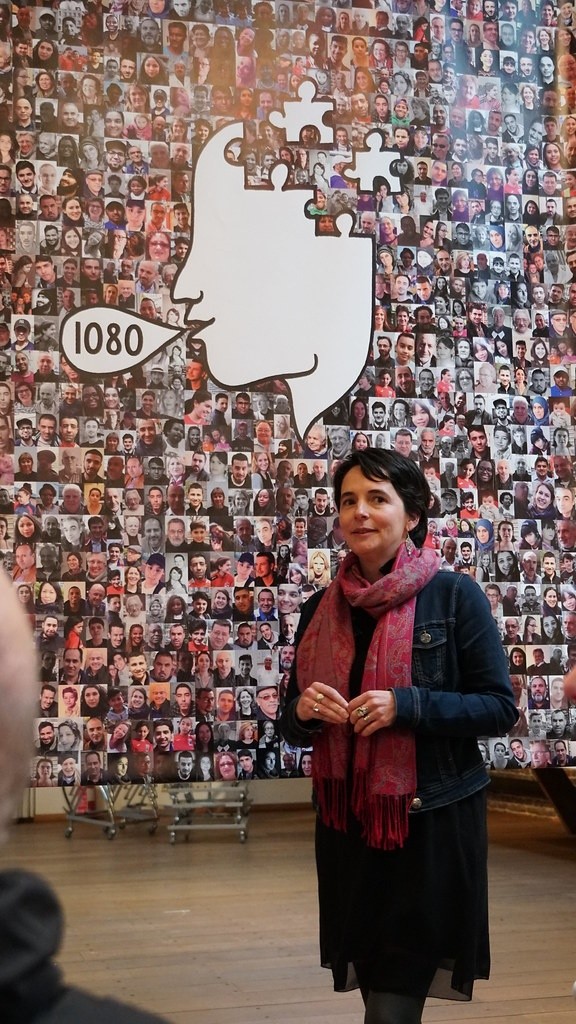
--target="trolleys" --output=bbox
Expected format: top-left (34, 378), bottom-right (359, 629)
top-left (165, 783), bottom-right (254, 843)
top-left (61, 785), bottom-right (159, 839)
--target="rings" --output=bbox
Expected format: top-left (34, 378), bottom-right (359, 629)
top-left (363, 716), bottom-right (371, 720)
top-left (313, 703), bottom-right (319, 712)
top-left (314, 694), bottom-right (324, 701)
top-left (356, 705), bottom-right (368, 717)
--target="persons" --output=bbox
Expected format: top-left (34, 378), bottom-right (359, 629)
top-left (126, 651), bottom-right (149, 685)
top-left (131, 721), bottom-right (152, 752)
top-left (276, 449), bottom-right (519, 1024)
top-left (494, 520), bottom-right (517, 550)
top-left (207, 619), bottom-right (232, 649)
top-left (0, 568), bottom-right (174, 1024)
top-left (0, 0), bottom-right (576, 785)
top-left (107, 722), bottom-right (128, 751)
top-left (144, 624), bottom-right (164, 649)
top-left (194, 722), bottom-right (213, 751)
top-left (440, 489), bottom-right (460, 519)
top-left (522, 586), bottom-right (542, 614)
top-left (216, 690), bottom-right (236, 720)
top-left (542, 551), bottom-right (560, 584)
top-left (234, 654), bottom-right (257, 685)
top-left (477, 491), bottom-right (497, 520)
